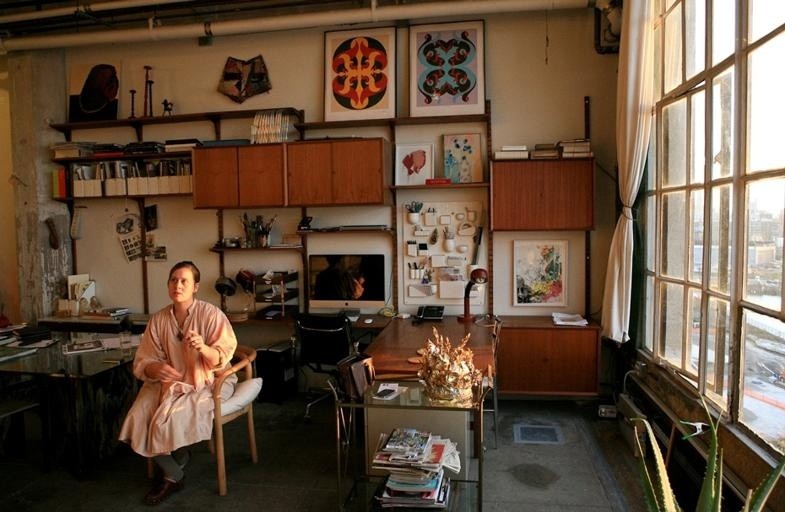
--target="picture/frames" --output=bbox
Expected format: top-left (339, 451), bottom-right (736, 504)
top-left (395, 142), bottom-right (433, 185)
top-left (512, 238), bottom-right (569, 308)
top-left (408, 18), bottom-right (486, 117)
top-left (322, 24), bottom-right (398, 120)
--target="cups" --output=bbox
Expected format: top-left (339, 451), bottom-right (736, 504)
top-left (119, 331), bottom-right (133, 357)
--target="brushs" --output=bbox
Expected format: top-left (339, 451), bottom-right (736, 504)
top-left (242, 212), bottom-right (278, 231)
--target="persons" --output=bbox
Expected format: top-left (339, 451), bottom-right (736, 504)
top-left (119, 261), bottom-right (238, 506)
top-left (315, 254), bottom-right (364, 300)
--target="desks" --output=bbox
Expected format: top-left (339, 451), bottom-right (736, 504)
top-left (0, 326), bottom-right (141, 474)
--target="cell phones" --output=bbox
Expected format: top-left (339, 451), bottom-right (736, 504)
top-left (376, 389), bottom-right (395, 399)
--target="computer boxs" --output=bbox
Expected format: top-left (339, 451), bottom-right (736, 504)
top-left (257, 340), bottom-right (295, 404)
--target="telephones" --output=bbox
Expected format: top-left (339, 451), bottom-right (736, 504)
top-left (412, 304), bottom-right (445, 325)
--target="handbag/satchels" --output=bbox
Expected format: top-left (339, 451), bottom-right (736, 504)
top-left (19, 326), bottom-right (52, 345)
top-left (338, 351), bottom-right (377, 403)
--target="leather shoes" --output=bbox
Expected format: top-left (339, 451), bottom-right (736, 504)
top-left (143, 478), bottom-right (185, 505)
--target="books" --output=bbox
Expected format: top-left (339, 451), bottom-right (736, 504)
top-left (51, 137), bottom-right (202, 199)
top-left (62, 335), bottom-right (141, 356)
top-left (66, 274), bottom-right (151, 325)
top-left (249, 113), bottom-right (300, 145)
top-left (0, 329), bottom-right (60, 361)
top-left (230, 311), bottom-right (249, 322)
top-left (372, 426), bottom-right (461, 508)
top-left (493, 137), bottom-right (595, 160)
top-left (552, 312), bottom-right (588, 326)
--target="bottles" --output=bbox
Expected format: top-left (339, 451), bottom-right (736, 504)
top-left (58, 288), bottom-right (66, 319)
top-left (65, 291), bottom-right (69, 316)
top-left (71, 294), bottom-right (79, 316)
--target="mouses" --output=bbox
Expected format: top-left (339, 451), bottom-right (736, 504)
top-left (365, 318), bottom-right (372, 323)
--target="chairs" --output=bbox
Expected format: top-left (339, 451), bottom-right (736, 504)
top-left (146, 345), bottom-right (259, 496)
top-left (296, 315), bottom-right (496, 512)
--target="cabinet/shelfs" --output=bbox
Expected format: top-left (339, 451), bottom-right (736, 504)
top-left (191, 144), bottom-right (287, 210)
top-left (50, 107), bottom-right (303, 203)
top-left (487, 158), bottom-right (596, 232)
top-left (489, 315), bottom-right (601, 410)
top-left (222, 207), bottom-right (305, 324)
top-left (288, 137), bottom-right (388, 208)
top-left (394, 121), bottom-right (493, 314)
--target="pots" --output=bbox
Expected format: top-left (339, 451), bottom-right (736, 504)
top-left (215, 251), bottom-right (237, 296)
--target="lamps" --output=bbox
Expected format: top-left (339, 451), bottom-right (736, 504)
top-left (456, 268), bottom-right (488, 320)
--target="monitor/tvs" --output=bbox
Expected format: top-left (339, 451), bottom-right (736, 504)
top-left (308, 254), bottom-right (385, 322)
